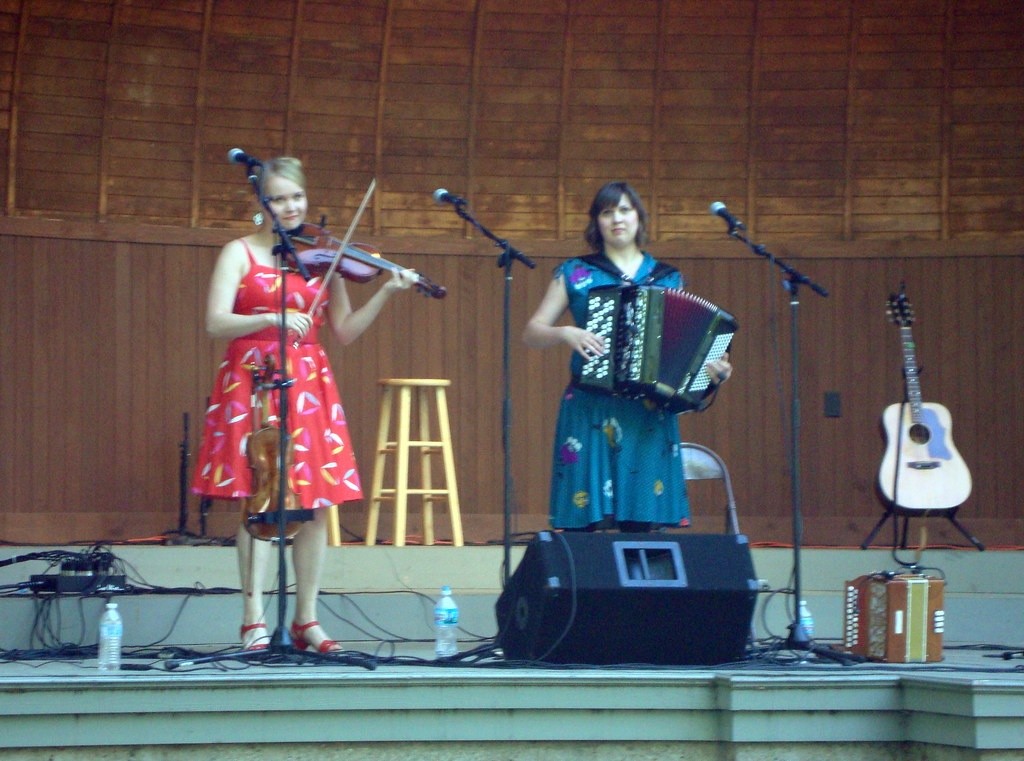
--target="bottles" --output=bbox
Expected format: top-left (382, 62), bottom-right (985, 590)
top-left (433, 586), bottom-right (459, 660)
top-left (97, 603), bottom-right (123, 671)
top-left (792, 600), bottom-right (815, 648)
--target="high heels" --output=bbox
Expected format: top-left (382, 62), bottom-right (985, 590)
top-left (291, 620), bottom-right (344, 654)
top-left (239, 622), bottom-right (270, 650)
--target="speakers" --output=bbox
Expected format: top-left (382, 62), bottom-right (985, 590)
top-left (496, 532), bottom-right (759, 666)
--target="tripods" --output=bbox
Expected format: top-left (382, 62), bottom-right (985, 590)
top-left (727, 223), bottom-right (871, 668)
top-left (163, 165), bottom-right (378, 669)
top-left (861, 368), bottom-right (986, 550)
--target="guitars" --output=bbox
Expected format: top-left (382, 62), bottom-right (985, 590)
top-left (873, 289), bottom-right (975, 516)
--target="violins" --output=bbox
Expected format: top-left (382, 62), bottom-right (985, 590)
top-left (281, 222), bottom-right (449, 299)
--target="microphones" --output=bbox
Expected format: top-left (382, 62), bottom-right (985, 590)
top-left (710, 202), bottom-right (747, 232)
top-left (226, 148), bottom-right (262, 166)
top-left (432, 187), bottom-right (468, 205)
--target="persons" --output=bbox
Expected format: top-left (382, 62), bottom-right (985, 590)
top-left (188, 157), bottom-right (420, 661)
top-left (523, 183), bottom-right (734, 535)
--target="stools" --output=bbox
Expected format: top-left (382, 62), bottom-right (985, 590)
top-left (365, 378), bottom-right (465, 547)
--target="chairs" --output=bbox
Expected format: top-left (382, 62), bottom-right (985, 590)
top-left (681, 442), bottom-right (739, 534)
